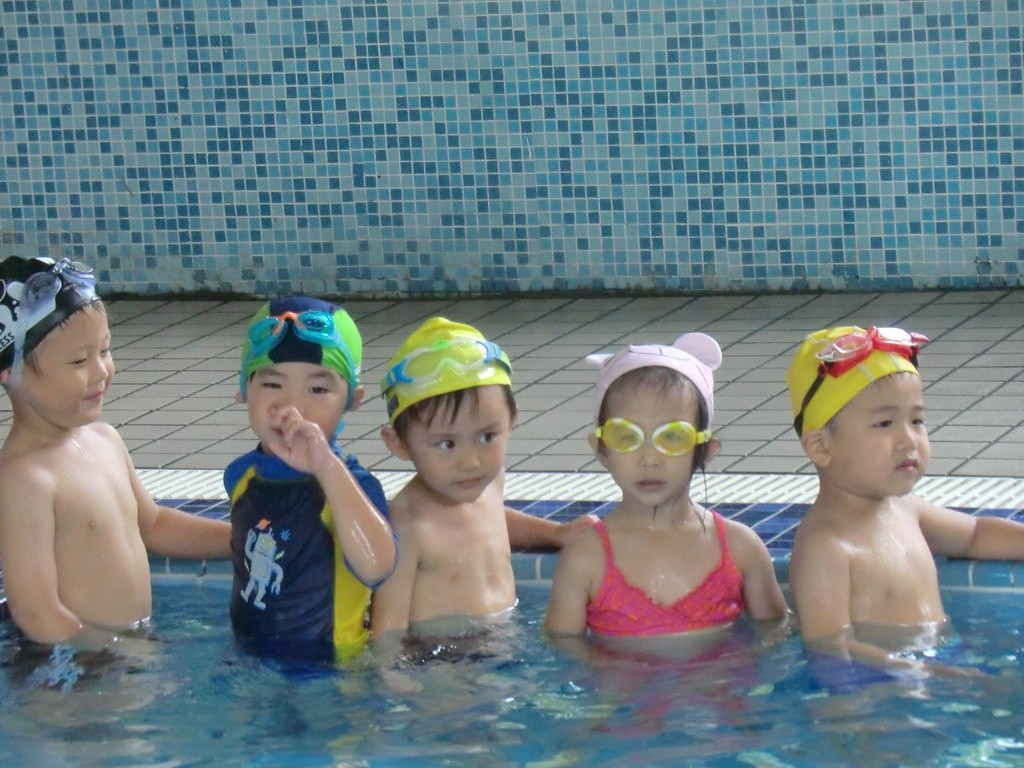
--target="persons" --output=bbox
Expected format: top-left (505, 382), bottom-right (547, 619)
top-left (0, 255), bottom-right (237, 720)
top-left (785, 326), bottom-right (1024, 678)
top-left (368, 315), bottom-right (603, 696)
top-left (220, 295), bottom-right (402, 678)
top-left (540, 344), bottom-right (789, 700)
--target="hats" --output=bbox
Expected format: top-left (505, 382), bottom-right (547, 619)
top-left (587, 332), bottom-right (722, 445)
top-left (787, 327), bottom-right (921, 441)
top-left (240, 298), bottom-right (362, 386)
top-left (0, 256), bottom-right (101, 372)
top-left (385, 318), bottom-right (511, 426)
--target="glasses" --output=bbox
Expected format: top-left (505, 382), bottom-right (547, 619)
top-left (795, 325), bottom-right (929, 435)
top-left (380, 336), bottom-right (512, 395)
top-left (7, 257), bottom-right (96, 389)
top-left (241, 311), bottom-right (355, 408)
top-left (594, 418), bottom-right (711, 455)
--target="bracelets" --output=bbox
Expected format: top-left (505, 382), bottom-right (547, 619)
top-left (97, 630), bottom-right (121, 656)
